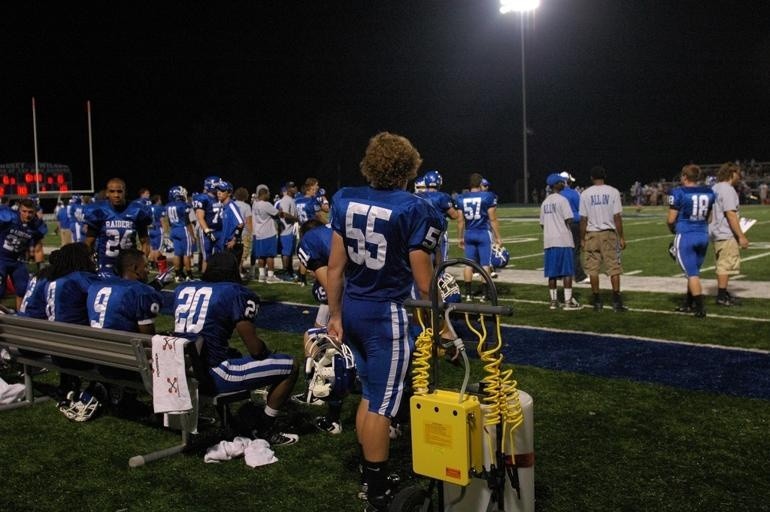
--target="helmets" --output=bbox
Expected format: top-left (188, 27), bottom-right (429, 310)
top-left (438, 271), bottom-right (462, 304)
top-left (415, 176), bottom-right (426, 188)
top-left (203, 175), bottom-right (221, 193)
top-left (168, 186), bottom-right (188, 202)
top-left (304, 335), bottom-right (358, 402)
top-left (423, 170), bottom-right (443, 188)
top-left (490, 243), bottom-right (510, 268)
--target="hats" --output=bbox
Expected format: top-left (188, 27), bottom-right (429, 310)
top-left (480, 178), bottom-right (490, 186)
top-left (215, 181), bottom-right (234, 192)
top-left (560, 171), bottom-right (576, 182)
top-left (546, 173), bottom-right (567, 185)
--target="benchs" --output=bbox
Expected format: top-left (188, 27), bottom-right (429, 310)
top-left (0, 313), bottom-right (261, 467)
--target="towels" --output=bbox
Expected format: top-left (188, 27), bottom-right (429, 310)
top-left (207, 434), bottom-right (282, 472)
top-left (147, 331), bottom-right (195, 426)
top-left (0, 375), bottom-right (31, 405)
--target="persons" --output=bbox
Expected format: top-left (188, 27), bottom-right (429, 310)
top-left (708, 163), bottom-right (748, 306)
top-left (531, 171), bottom-right (590, 310)
top-left (1, 173), bottom-right (355, 450)
top-left (630, 159), bottom-right (770, 207)
top-left (667, 164), bottom-right (715, 318)
top-left (321, 135), bottom-right (457, 501)
top-left (579, 166), bottom-right (629, 313)
top-left (417, 170), bottom-right (501, 302)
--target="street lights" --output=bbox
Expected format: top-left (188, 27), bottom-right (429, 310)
top-left (500, 1), bottom-right (540, 205)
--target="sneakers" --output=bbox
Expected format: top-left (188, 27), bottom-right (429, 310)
top-left (290, 393), bottom-right (326, 406)
top-left (357, 474), bottom-right (401, 501)
top-left (549, 300), bottom-right (559, 310)
top-left (240, 268), bottom-right (307, 287)
top-left (313, 415), bottom-right (343, 435)
top-left (673, 296), bottom-right (742, 319)
top-left (257, 429), bottom-right (300, 446)
top-left (149, 267), bottom-right (202, 283)
top-left (562, 299), bottom-right (584, 311)
